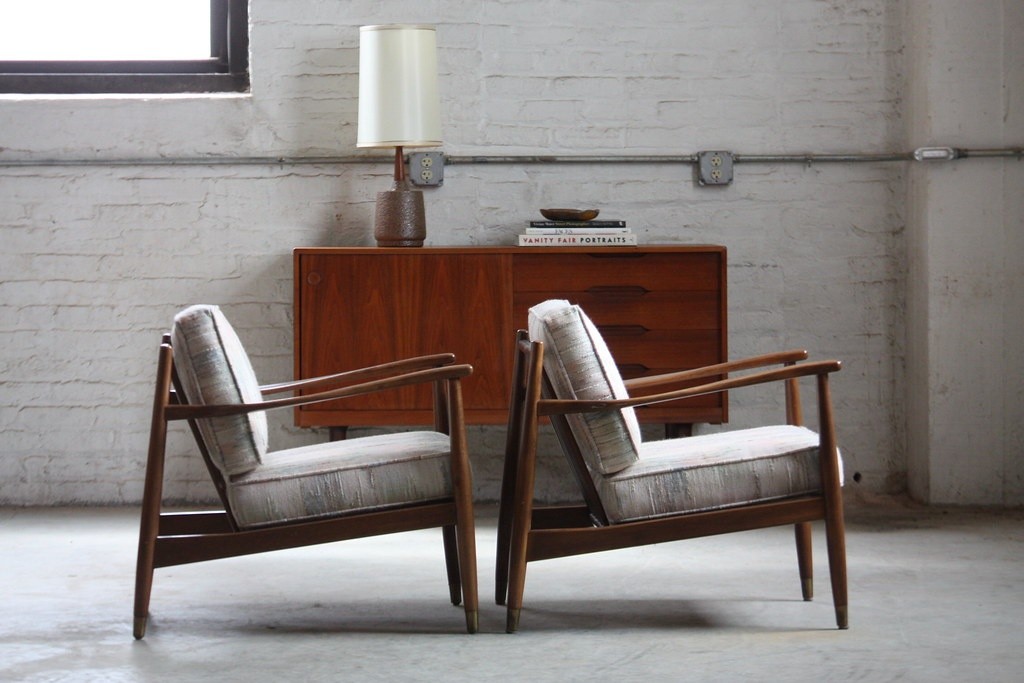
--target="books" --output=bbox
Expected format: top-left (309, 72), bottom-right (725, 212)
top-left (519, 220), bottom-right (637, 247)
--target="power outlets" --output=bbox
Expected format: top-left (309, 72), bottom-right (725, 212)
top-left (698, 151), bottom-right (733, 185)
top-left (409, 152), bottom-right (444, 185)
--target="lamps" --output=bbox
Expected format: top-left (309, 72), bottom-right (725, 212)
top-left (358, 25), bottom-right (440, 246)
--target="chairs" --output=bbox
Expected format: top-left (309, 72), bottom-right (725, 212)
top-left (497, 297), bottom-right (848, 636)
top-left (132, 302), bottom-right (478, 639)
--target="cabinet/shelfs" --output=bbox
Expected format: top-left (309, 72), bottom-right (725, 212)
top-left (293, 244), bottom-right (730, 438)
